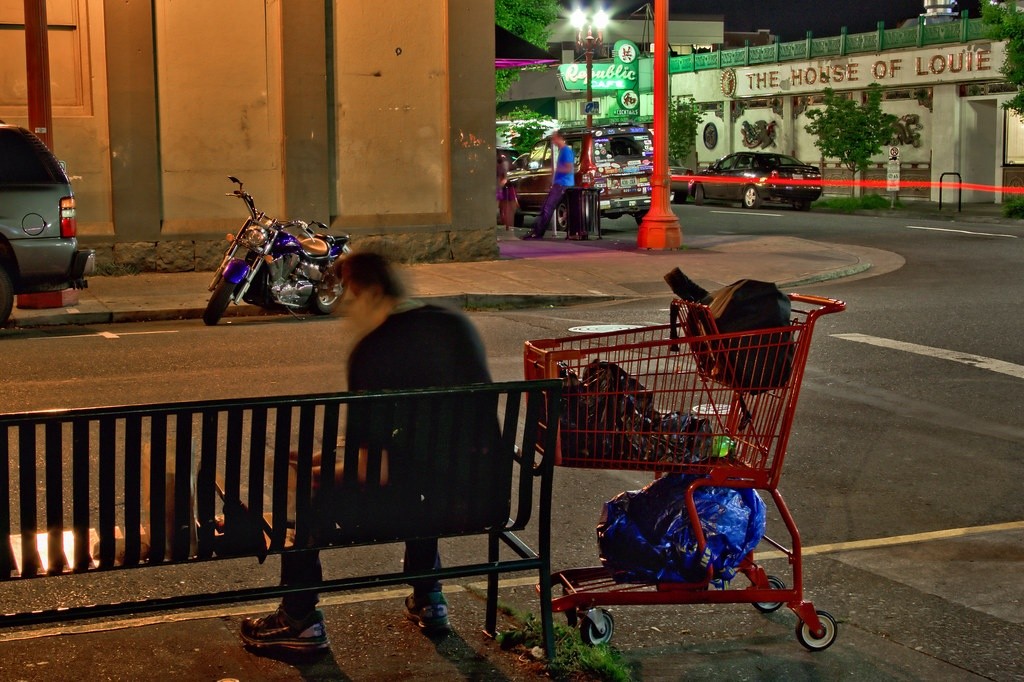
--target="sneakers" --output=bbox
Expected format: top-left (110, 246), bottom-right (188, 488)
top-left (240, 605), bottom-right (329, 648)
top-left (403, 593), bottom-right (450, 628)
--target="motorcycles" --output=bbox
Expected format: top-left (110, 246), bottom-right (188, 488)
top-left (201, 173), bottom-right (356, 326)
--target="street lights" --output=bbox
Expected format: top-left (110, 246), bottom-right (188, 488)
top-left (569, 5), bottom-right (610, 188)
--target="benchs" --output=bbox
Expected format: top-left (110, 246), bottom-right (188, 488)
top-left (1, 374), bottom-right (565, 680)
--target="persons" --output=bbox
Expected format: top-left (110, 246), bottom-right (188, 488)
top-left (240, 255), bottom-right (507, 658)
top-left (497, 130), bottom-right (575, 240)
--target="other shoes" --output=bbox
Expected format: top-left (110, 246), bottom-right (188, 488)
top-left (519, 230), bottom-right (542, 240)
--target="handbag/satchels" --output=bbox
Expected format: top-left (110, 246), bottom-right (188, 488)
top-left (663, 266), bottom-right (795, 388)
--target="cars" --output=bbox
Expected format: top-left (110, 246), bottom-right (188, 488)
top-left (689, 152), bottom-right (823, 211)
top-left (668, 155), bottom-right (695, 204)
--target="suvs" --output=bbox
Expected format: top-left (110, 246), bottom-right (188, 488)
top-left (0, 118), bottom-right (96, 330)
top-left (498, 122), bottom-right (656, 232)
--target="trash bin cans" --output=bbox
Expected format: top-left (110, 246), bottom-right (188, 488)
top-left (563, 186), bottom-right (602, 240)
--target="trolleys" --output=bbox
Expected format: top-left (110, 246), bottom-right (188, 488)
top-left (522, 287), bottom-right (839, 652)
